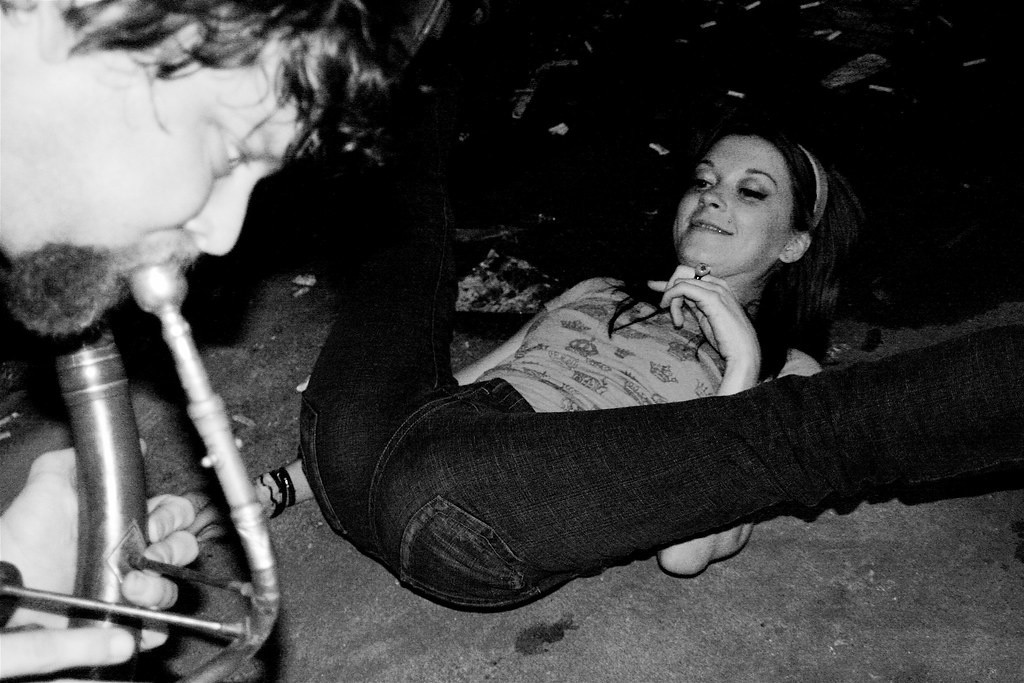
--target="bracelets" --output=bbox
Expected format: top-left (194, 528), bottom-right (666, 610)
top-left (258, 465), bottom-right (299, 518)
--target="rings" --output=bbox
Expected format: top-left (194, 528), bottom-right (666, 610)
top-left (695, 262), bottom-right (710, 279)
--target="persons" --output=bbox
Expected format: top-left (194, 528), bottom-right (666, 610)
top-left (0, 0), bottom-right (407, 683)
top-left (174, 0), bottom-right (1024, 611)
